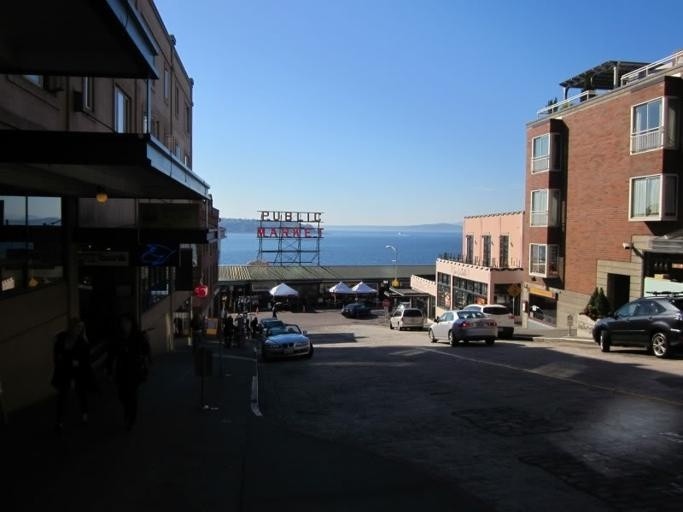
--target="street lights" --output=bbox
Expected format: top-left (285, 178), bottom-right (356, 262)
top-left (385, 244), bottom-right (398, 281)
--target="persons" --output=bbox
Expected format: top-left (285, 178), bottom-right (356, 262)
top-left (48, 315), bottom-right (101, 433)
top-left (98, 310), bottom-right (154, 434)
top-left (272, 304), bottom-right (278, 320)
top-left (213, 310), bottom-right (259, 352)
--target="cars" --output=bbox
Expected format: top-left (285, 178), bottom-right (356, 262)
top-left (341, 303), bottom-right (371, 317)
top-left (428, 309), bottom-right (498, 345)
top-left (261, 317), bottom-right (313, 363)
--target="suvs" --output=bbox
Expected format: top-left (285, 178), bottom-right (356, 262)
top-left (591, 296), bottom-right (683, 359)
top-left (462, 302), bottom-right (514, 339)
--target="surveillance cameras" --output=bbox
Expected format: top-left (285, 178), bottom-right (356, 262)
top-left (622, 242), bottom-right (631, 250)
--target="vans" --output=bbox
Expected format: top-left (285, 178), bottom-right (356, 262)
top-left (390, 307), bottom-right (424, 331)
